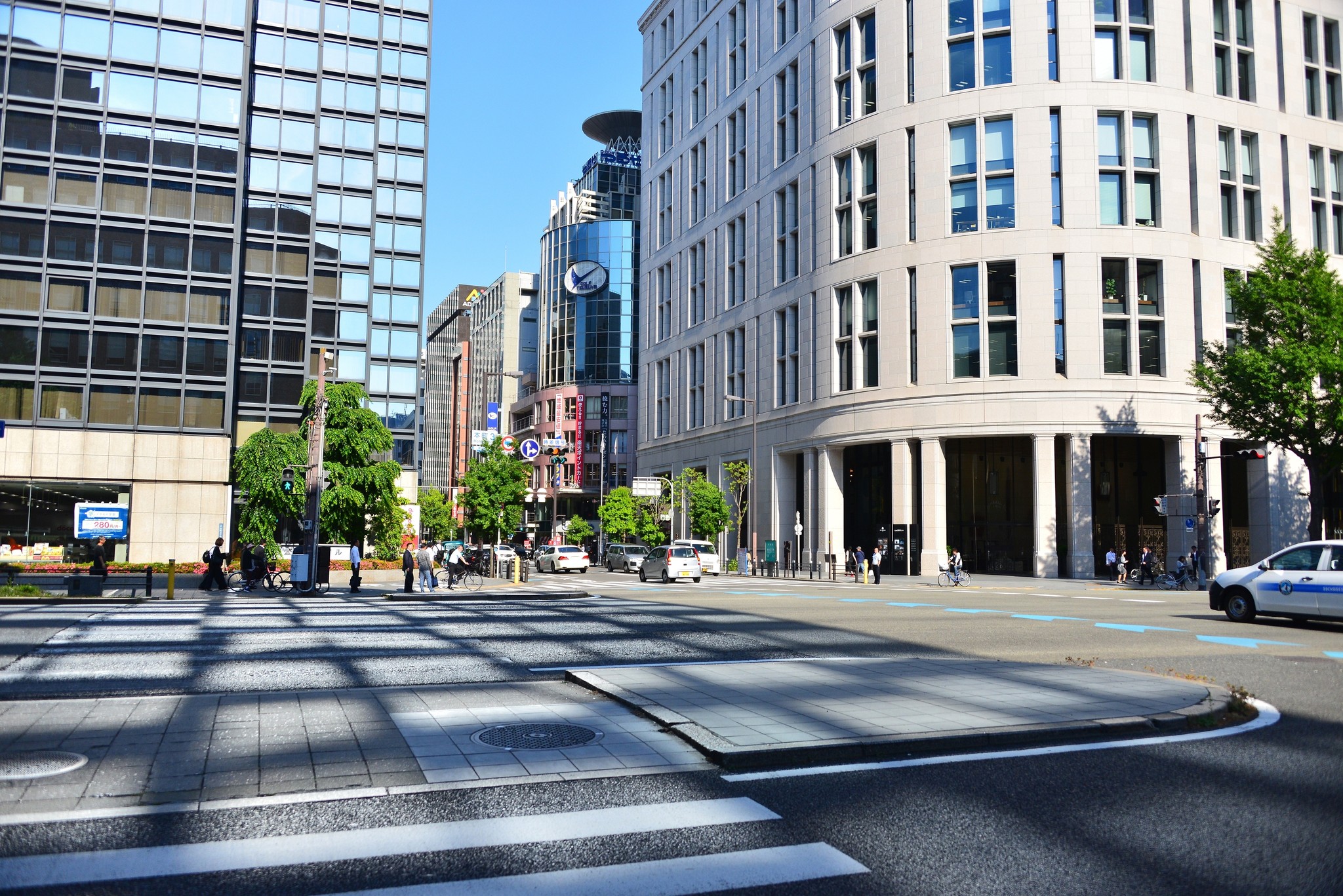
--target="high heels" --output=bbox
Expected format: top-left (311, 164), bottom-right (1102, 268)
top-left (224, 586), bottom-right (230, 590)
top-left (205, 588), bottom-right (213, 591)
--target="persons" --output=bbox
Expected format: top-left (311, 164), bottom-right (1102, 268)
top-left (432, 540), bottom-right (444, 568)
top-left (447, 545), bottom-right (470, 591)
top-left (854, 547), bottom-right (864, 574)
top-left (845, 546), bottom-right (857, 577)
top-left (945, 548), bottom-right (963, 587)
top-left (402, 542), bottom-right (415, 593)
top-left (416, 543), bottom-right (436, 593)
top-left (292, 538), bottom-right (304, 555)
top-left (197, 537), bottom-right (232, 592)
top-left (92, 536), bottom-right (108, 584)
top-left (349, 539), bottom-right (363, 594)
top-left (423, 542), bottom-right (434, 588)
top-left (249, 539), bottom-right (278, 590)
top-left (1105, 545), bottom-right (1199, 594)
top-left (872, 548), bottom-right (882, 584)
top-left (239, 543), bottom-right (265, 593)
top-left (579, 544), bottom-right (585, 552)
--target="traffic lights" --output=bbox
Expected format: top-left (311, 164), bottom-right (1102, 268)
top-left (542, 446), bottom-right (568, 455)
top-left (550, 455), bottom-right (567, 464)
top-left (1209, 499), bottom-right (1220, 516)
top-left (1153, 497), bottom-right (1162, 514)
top-left (550, 515), bottom-right (553, 526)
top-left (322, 468), bottom-right (331, 491)
top-left (281, 468), bottom-right (295, 492)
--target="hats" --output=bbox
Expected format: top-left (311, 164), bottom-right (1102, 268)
top-left (246, 543), bottom-right (256, 549)
top-left (856, 546), bottom-right (862, 549)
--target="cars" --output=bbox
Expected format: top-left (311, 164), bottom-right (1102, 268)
top-left (639, 545), bottom-right (702, 584)
top-left (1209, 539), bottom-right (1343, 627)
top-left (533, 545), bottom-right (554, 567)
top-left (418, 538), bottom-right (535, 577)
top-left (536, 545), bottom-right (590, 574)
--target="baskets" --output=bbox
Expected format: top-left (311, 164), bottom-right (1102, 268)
top-left (267, 562), bottom-right (276, 571)
top-left (470, 565), bottom-right (476, 572)
top-left (939, 567), bottom-right (948, 571)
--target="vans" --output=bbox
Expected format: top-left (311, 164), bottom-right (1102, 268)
top-left (607, 544), bottom-right (651, 573)
top-left (603, 542), bottom-right (644, 569)
top-left (672, 539), bottom-right (720, 576)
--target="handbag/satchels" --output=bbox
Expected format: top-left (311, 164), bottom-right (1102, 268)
top-left (431, 573), bottom-right (438, 587)
top-left (349, 576), bottom-right (362, 586)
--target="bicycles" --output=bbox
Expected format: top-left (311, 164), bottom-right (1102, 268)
top-left (938, 562), bottom-right (973, 588)
top-left (435, 562), bottom-right (483, 591)
top-left (228, 557), bottom-right (283, 593)
top-left (1130, 560), bottom-right (1163, 582)
top-left (1156, 565), bottom-right (1200, 591)
top-left (272, 571), bottom-right (321, 594)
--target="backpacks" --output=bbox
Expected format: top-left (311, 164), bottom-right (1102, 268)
top-left (203, 550), bottom-right (210, 563)
top-left (435, 545), bottom-right (444, 559)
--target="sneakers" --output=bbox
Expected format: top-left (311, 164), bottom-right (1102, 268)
top-left (244, 587), bottom-right (252, 593)
top-left (251, 585), bottom-right (258, 589)
top-left (844, 572), bottom-right (881, 583)
top-left (254, 579), bottom-right (262, 583)
top-left (955, 583), bottom-right (958, 586)
top-left (269, 585), bottom-right (278, 590)
top-left (954, 575), bottom-right (956, 578)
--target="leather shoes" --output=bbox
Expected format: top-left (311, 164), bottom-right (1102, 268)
top-left (218, 587), bottom-right (225, 590)
top-left (457, 577), bottom-right (465, 579)
top-left (198, 585), bottom-right (206, 589)
top-left (447, 586), bottom-right (454, 590)
top-left (356, 589), bottom-right (361, 592)
top-left (1107, 575), bottom-right (1218, 587)
top-left (350, 590), bottom-right (360, 593)
